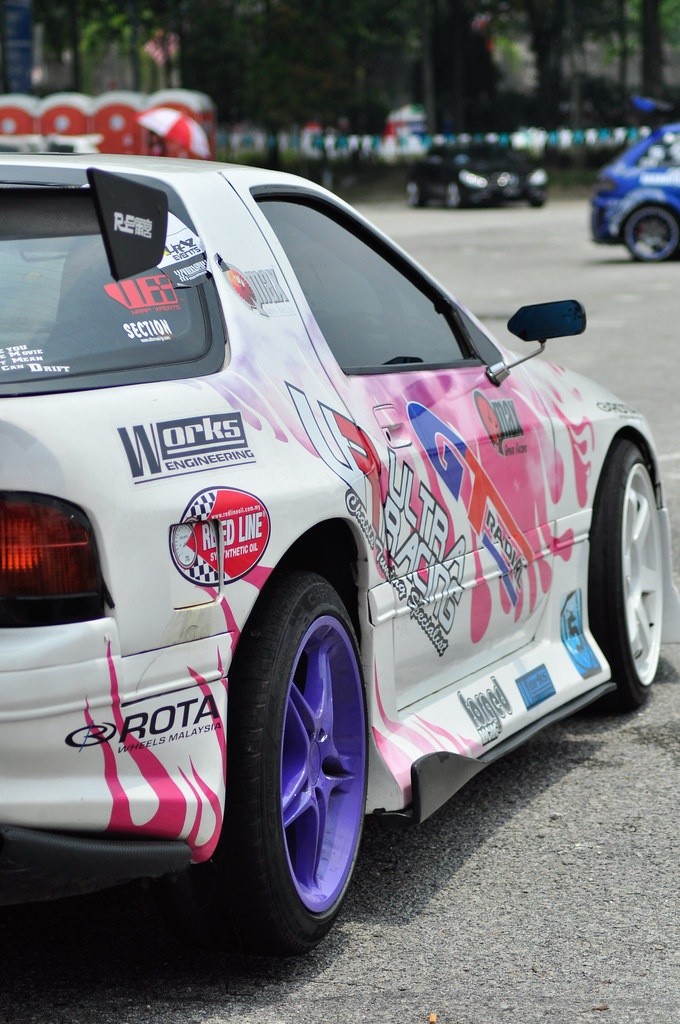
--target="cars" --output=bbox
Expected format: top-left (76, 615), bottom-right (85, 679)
top-left (588, 125), bottom-right (680, 264)
top-left (0, 149), bottom-right (668, 957)
top-left (404, 143), bottom-right (549, 213)
